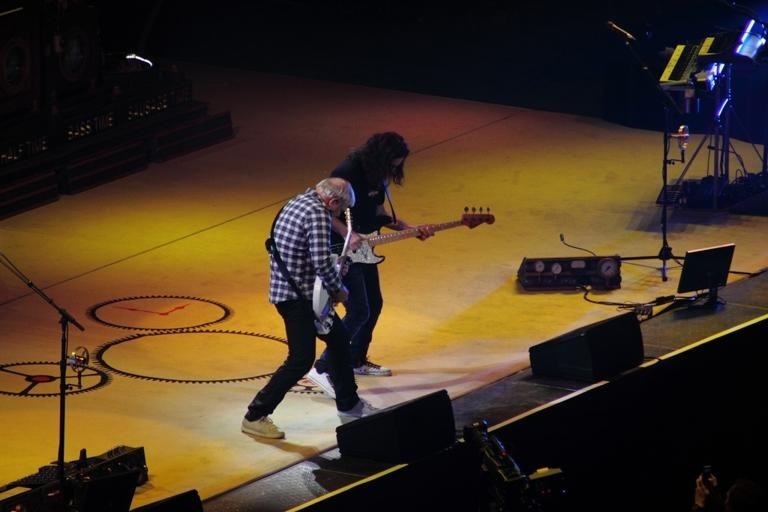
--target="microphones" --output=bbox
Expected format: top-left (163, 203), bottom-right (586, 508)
top-left (606, 20), bottom-right (636, 41)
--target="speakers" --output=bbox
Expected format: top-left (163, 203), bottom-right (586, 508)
top-left (0, 0), bottom-right (49, 138)
top-left (528, 312), bottom-right (643, 384)
top-left (335, 389), bottom-right (456, 464)
top-left (49, 2), bottom-right (105, 106)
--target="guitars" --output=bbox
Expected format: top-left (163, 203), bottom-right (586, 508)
top-left (346, 207), bottom-right (495, 264)
top-left (313, 208), bottom-right (353, 335)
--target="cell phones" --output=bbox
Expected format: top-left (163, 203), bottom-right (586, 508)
top-left (702, 466), bottom-right (714, 490)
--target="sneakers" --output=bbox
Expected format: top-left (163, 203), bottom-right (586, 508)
top-left (305, 367), bottom-right (337, 398)
top-left (240, 414), bottom-right (286, 440)
top-left (335, 398), bottom-right (381, 419)
top-left (351, 363), bottom-right (392, 377)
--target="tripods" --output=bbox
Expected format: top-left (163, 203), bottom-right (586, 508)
top-left (675, 63), bottom-right (750, 210)
top-left (620, 40), bottom-right (685, 281)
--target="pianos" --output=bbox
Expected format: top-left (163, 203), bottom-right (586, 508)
top-left (659, 20), bottom-right (766, 89)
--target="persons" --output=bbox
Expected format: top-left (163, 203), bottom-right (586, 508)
top-left (242, 177), bottom-right (381, 439)
top-left (694, 473), bottom-right (768, 512)
top-left (306, 132), bottom-right (434, 398)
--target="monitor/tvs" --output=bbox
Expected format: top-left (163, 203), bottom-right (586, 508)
top-left (679, 245), bottom-right (735, 308)
